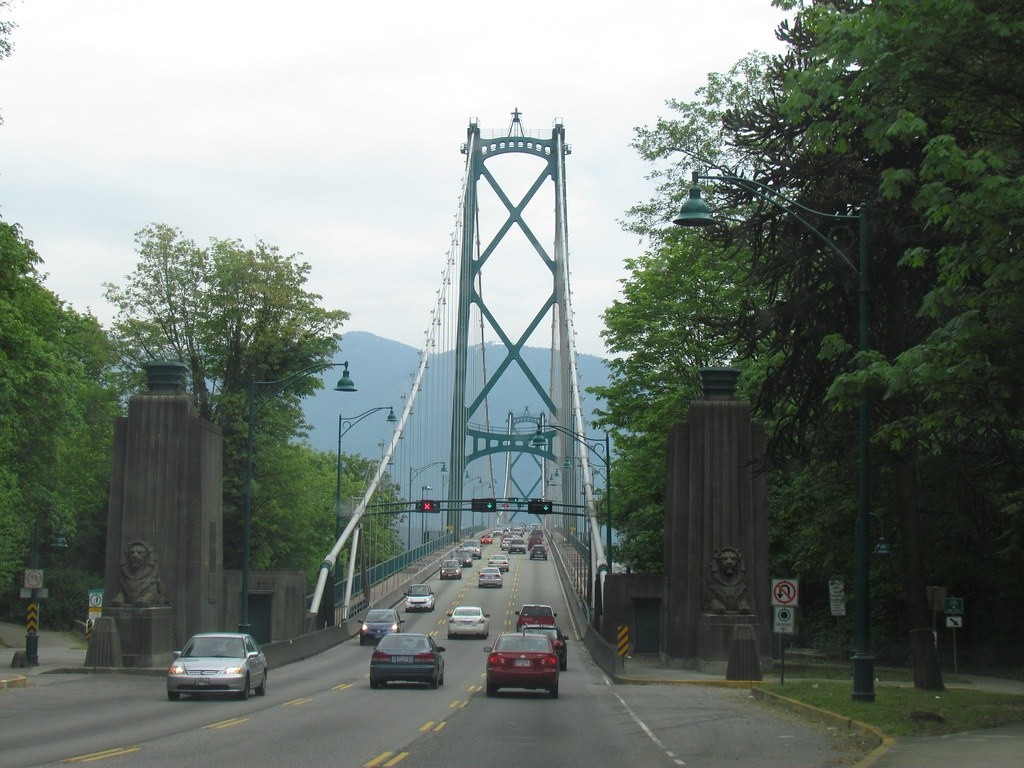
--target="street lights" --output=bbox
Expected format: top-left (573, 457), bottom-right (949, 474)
top-left (850, 512), bottom-right (895, 675)
top-left (670, 170), bottom-right (876, 704)
top-left (407, 461), bottom-right (449, 549)
top-left (441, 470), bottom-right (470, 536)
top-left (239, 360), bottom-right (359, 634)
top-left (472, 482), bottom-right (492, 526)
top-left (24, 508), bottom-right (69, 666)
top-left (529, 411), bottom-right (613, 573)
top-left (333, 405), bottom-right (400, 607)
top-left (562, 455), bottom-right (602, 539)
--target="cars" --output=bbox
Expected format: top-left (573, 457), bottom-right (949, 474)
top-left (439, 560), bottom-right (464, 580)
top-left (166, 633), bottom-right (268, 701)
top-left (482, 633), bottom-right (564, 700)
top-left (518, 622), bottom-right (570, 671)
top-left (450, 522), bottom-right (550, 572)
top-left (404, 583), bottom-right (435, 612)
top-left (515, 604), bottom-right (558, 633)
top-left (447, 606), bottom-right (491, 639)
top-left (478, 566), bottom-right (504, 588)
top-left (369, 632), bottom-right (446, 689)
top-left (357, 609), bottom-right (406, 646)
